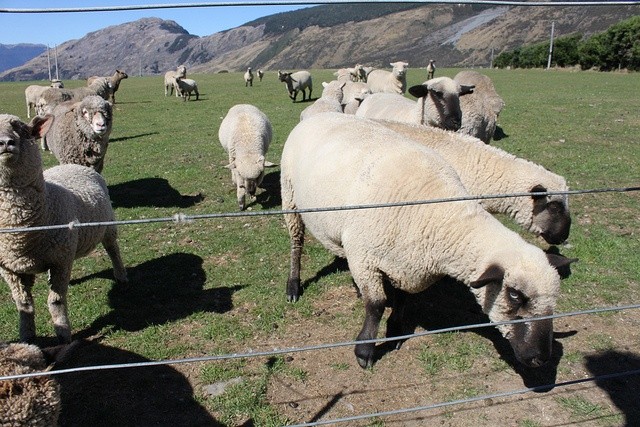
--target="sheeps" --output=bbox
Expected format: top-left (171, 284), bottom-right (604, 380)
top-left (37, 76), bottom-right (111, 151)
top-left (300, 80), bottom-right (346, 118)
top-left (333, 64), bottom-right (363, 81)
top-left (366, 61), bottom-right (409, 96)
top-left (426, 57), bottom-right (436, 80)
top-left (173, 76), bottom-right (199, 101)
top-left (257, 70), bottom-right (264, 81)
top-left (367, 118), bottom-right (570, 248)
top-left (218, 104), bottom-right (279, 210)
top-left (46, 95), bottom-right (112, 174)
top-left (276, 68), bottom-right (312, 102)
top-left (164, 65), bottom-right (186, 97)
top-left (25, 83), bottom-right (65, 118)
top-left (332, 80), bottom-right (373, 115)
top-left (280, 112), bottom-right (579, 369)
top-left (244, 68), bottom-right (253, 88)
top-left (356, 76), bottom-right (476, 131)
top-left (362, 66), bottom-right (374, 77)
top-left (0, 115), bottom-right (129, 341)
top-left (0, 341), bottom-right (62, 426)
top-left (453, 71), bottom-right (503, 145)
top-left (88, 69), bottom-right (128, 104)
